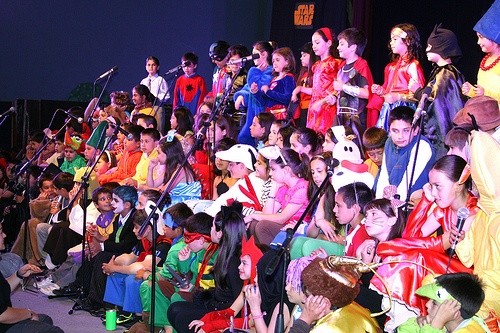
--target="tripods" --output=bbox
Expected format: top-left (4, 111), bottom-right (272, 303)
top-left (47, 129), bottom-right (122, 314)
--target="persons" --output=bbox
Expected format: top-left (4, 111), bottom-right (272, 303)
top-left (0, 157), bottom-right (327, 333)
top-left (140, 28), bottom-right (341, 233)
top-left (307, 0), bottom-right (500, 333)
top-left (26, 56), bottom-right (169, 189)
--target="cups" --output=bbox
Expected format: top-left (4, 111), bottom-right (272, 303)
top-left (105, 309), bottom-right (117, 331)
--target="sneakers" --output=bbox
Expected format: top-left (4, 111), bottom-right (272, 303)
top-left (40, 282), bottom-right (60, 296)
top-left (33, 277), bottom-right (53, 288)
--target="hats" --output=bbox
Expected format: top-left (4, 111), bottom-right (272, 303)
top-left (301, 254), bottom-right (436, 318)
top-left (416, 279), bottom-right (461, 307)
top-left (86, 121), bottom-right (108, 151)
top-left (473, 0), bottom-right (500, 45)
top-left (215, 144), bottom-right (257, 172)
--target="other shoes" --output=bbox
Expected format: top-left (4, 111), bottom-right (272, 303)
top-left (52, 285), bottom-right (77, 294)
top-left (116, 310), bottom-right (136, 324)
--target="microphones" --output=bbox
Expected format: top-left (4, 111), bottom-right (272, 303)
top-left (0, 106), bottom-right (15, 118)
top-left (63, 110), bottom-right (83, 124)
top-left (96, 65), bottom-right (118, 80)
top-left (325, 158), bottom-right (339, 168)
top-left (109, 122), bottom-right (134, 141)
top-left (414, 86), bottom-right (430, 124)
top-left (232, 53), bottom-right (261, 64)
top-left (167, 60), bottom-right (191, 74)
top-left (448, 207), bottom-right (468, 255)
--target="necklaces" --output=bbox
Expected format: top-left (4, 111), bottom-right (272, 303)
top-left (480, 52), bottom-right (500, 71)
top-left (342, 64), bottom-right (354, 72)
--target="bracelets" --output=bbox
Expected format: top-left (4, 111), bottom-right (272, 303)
top-left (268, 195), bottom-right (275, 199)
top-left (28, 309), bottom-right (34, 319)
top-left (342, 84), bottom-right (360, 98)
top-left (332, 92), bottom-right (340, 98)
top-left (313, 215), bottom-right (320, 228)
top-left (253, 311), bottom-right (266, 319)
top-left (16, 271), bottom-right (25, 279)
